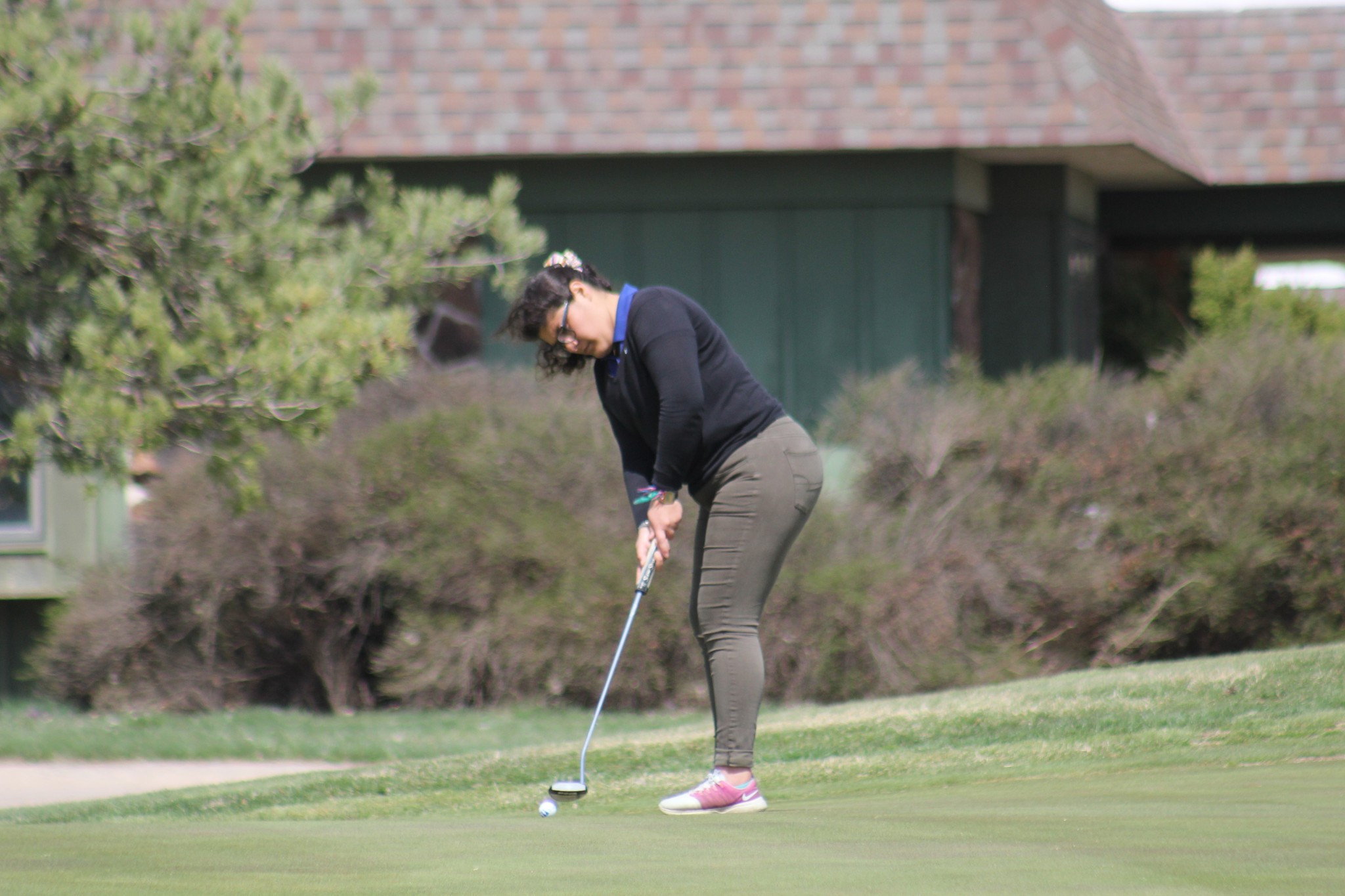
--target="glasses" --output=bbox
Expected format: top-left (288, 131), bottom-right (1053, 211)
top-left (550, 302), bottom-right (576, 359)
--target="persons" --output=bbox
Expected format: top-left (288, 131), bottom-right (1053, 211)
top-left (504, 250), bottom-right (825, 813)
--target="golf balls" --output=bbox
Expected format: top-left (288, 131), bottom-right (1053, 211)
top-left (538, 800), bottom-right (557, 818)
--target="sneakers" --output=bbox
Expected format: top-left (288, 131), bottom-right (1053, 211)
top-left (658, 770), bottom-right (768, 815)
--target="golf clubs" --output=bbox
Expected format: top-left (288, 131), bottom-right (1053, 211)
top-left (549, 537), bottom-right (659, 803)
